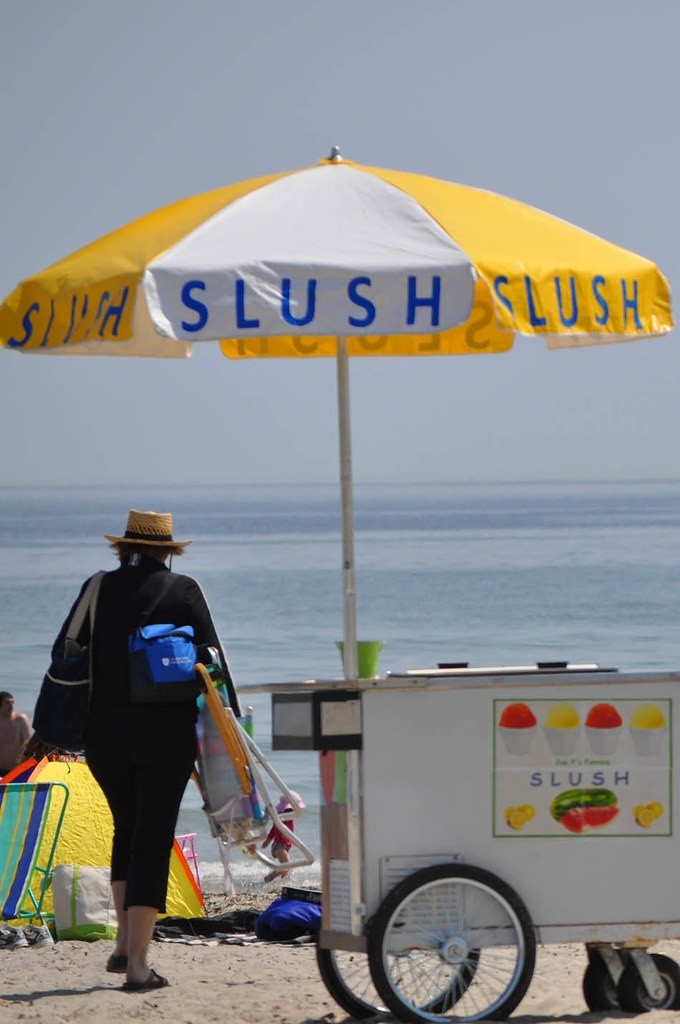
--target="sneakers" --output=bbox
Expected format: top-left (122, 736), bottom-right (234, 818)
top-left (0, 923), bottom-right (29, 949)
top-left (22, 923), bottom-right (54, 948)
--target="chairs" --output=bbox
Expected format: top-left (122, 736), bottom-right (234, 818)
top-left (176, 832), bottom-right (200, 888)
top-left (1, 782), bottom-right (69, 944)
top-left (196, 646), bottom-right (315, 871)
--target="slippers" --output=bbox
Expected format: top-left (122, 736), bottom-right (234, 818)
top-left (122, 968), bottom-right (169, 990)
top-left (106, 954), bottom-right (128, 973)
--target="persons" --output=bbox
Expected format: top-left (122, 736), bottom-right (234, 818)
top-left (262, 791), bottom-right (305, 882)
top-left (0, 691), bottom-right (33, 777)
top-left (33, 509), bottom-right (241, 989)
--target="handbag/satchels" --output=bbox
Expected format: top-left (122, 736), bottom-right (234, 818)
top-left (52, 864), bottom-right (118, 941)
top-left (31, 569), bottom-right (107, 753)
top-left (125, 572), bottom-right (205, 703)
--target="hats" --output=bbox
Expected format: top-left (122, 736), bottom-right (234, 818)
top-left (275, 791), bottom-right (306, 813)
top-left (103, 509), bottom-right (193, 546)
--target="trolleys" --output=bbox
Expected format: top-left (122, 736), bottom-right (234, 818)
top-left (230, 662), bottom-right (680, 1024)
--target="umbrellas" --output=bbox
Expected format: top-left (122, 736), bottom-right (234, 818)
top-left (0, 145), bottom-right (676, 680)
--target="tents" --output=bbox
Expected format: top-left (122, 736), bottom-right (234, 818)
top-left (0, 753), bottom-right (207, 930)
